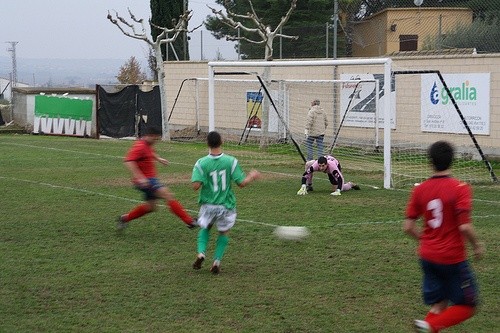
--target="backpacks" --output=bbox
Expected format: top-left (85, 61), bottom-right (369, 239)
top-left (249, 116), bottom-right (261, 128)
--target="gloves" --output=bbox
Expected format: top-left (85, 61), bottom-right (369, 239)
top-left (297, 184), bottom-right (308, 195)
top-left (304, 129), bottom-right (309, 138)
top-left (330, 189), bottom-right (341, 196)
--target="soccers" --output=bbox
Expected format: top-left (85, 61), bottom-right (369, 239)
top-left (275, 225), bottom-right (308, 241)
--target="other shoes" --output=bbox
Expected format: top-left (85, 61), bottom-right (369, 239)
top-left (210, 260), bottom-right (220, 273)
top-left (192, 253), bottom-right (206, 269)
top-left (414, 320), bottom-right (432, 333)
top-left (351, 182), bottom-right (360, 190)
top-left (306, 186), bottom-right (313, 191)
top-left (117, 214), bottom-right (125, 231)
top-left (186, 217), bottom-right (199, 230)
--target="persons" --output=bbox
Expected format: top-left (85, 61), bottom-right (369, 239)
top-left (296, 155), bottom-right (361, 196)
top-left (192, 131), bottom-right (260, 275)
top-left (305, 99), bottom-right (328, 161)
top-left (117, 126), bottom-right (200, 231)
top-left (403, 142), bottom-right (484, 333)
